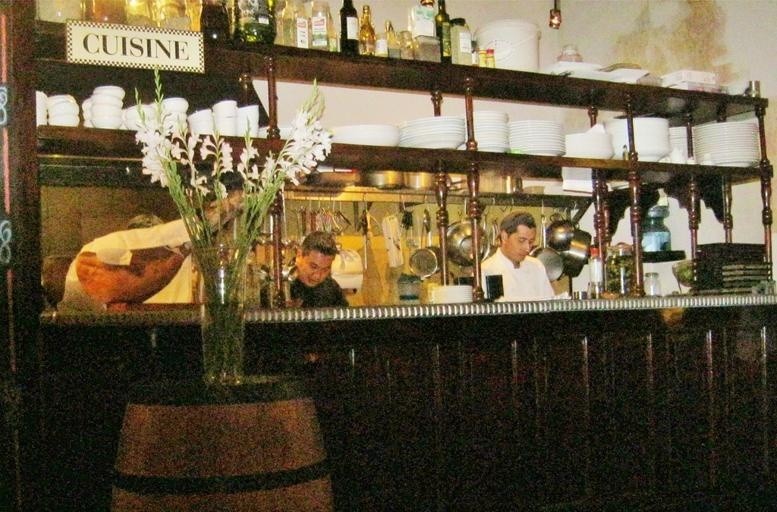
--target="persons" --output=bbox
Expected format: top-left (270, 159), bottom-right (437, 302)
top-left (472, 210), bottom-right (555, 301)
top-left (39, 254), bottom-right (72, 315)
top-left (259, 230), bottom-right (349, 307)
top-left (128, 213), bottom-right (163, 230)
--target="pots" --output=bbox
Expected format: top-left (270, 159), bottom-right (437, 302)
top-left (417, 199), bottom-right (592, 281)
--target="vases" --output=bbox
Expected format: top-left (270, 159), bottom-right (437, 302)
top-left (131, 67), bottom-right (332, 388)
top-left (190, 248), bottom-right (252, 389)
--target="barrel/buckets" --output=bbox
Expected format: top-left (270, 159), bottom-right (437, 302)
top-left (473, 19), bottom-right (540, 73)
top-left (110, 376), bottom-right (335, 512)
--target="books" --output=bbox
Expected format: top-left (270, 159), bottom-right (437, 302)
top-left (691, 242), bottom-right (772, 296)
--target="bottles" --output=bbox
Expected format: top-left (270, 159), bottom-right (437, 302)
top-left (641, 206), bottom-right (672, 253)
top-left (127, 2), bottom-right (151, 25)
top-left (605, 244), bottom-right (636, 296)
top-left (599, 281), bottom-right (605, 299)
top-left (200, 0), bottom-right (230, 39)
top-left (643, 272), bottom-right (662, 298)
top-left (241, 1), bottom-right (275, 44)
top-left (361, 5), bottom-right (376, 57)
top-left (452, 17), bottom-right (472, 66)
top-left (387, 22), bottom-right (401, 59)
top-left (281, 1), bottom-right (295, 46)
top-left (587, 282), bottom-right (597, 299)
top-left (185, 0), bottom-right (204, 32)
top-left (377, 33), bottom-right (389, 58)
top-left (340, 0), bottom-right (360, 57)
top-left (313, 1), bottom-right (335, 52)
top-left (35, 0), bottom-right (88, 23)
top-left (436, 1), bottom-right (451, 62)
top-left (90, 1), bottom-right (125, 22)
top-left (227, 1), bottom-right (243, 40)
top-left (297, 1), bottom-right (310, 48)
top-left (555, 43), bottom-right (583, 61)
top-left (401, 31), bottom-right (415, 59)
top-left (152, 1), bottom-right (182, 29)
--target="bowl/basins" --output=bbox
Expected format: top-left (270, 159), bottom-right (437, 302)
top-left (331, 123), bottom-right (402, 147)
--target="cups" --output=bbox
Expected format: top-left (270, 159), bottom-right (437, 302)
top-left (36, 84), bottom-right (261, 136)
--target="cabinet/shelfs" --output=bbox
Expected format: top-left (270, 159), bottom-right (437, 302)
top-left (0, 0), bottom-right (777, 327)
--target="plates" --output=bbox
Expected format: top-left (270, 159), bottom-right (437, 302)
top-left (398, 109), bottom-right (568, 160)
top-left (545, 62), bottom-right (646, 84)
top-left (602, 116), bottom-right (671, 162)
top-left (668, 120), bottom-right (763, 165)
